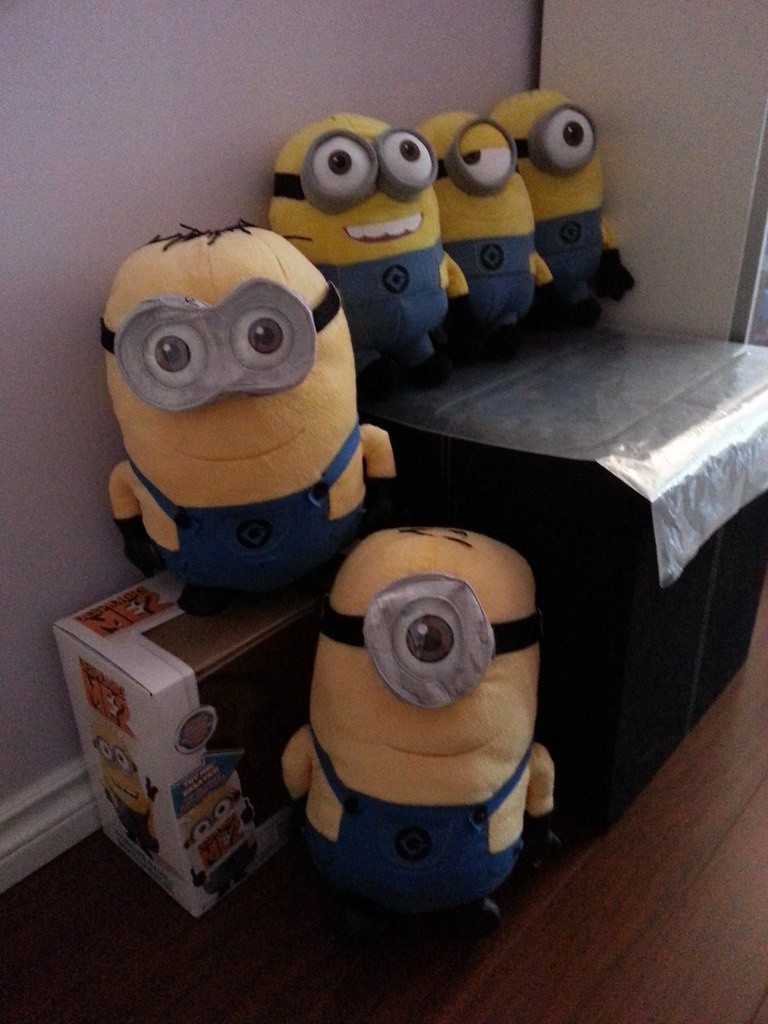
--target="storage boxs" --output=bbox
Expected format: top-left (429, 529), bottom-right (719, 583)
top-left (50, 550), bottom-right (346, 916)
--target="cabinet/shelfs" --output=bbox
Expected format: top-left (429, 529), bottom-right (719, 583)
top-left (364, 320), bottom-right (761, 832)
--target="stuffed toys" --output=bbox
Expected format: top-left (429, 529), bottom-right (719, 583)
top-left (101, 219), bottom-right (397, 616)
top-left (267, 114), bottom-right (449, 398)
top-left (283, 527), bottom-right (555, 944)
top-left (490, 92), bottom-right (634, 345)
top-left (419, 111), bottom-right (536, 363)
